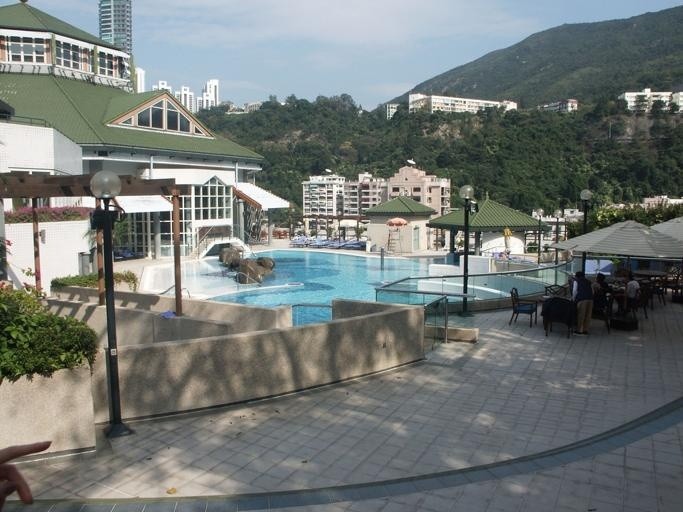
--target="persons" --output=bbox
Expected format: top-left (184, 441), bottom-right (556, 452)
top-left (571, 271), bottom-right (593, 335)
top-left (591, 273), bottom-right (609, 313)
top-left (614, 275), bottom-right (641, 313)
top-left (0, 442), bottom-right (52, 512)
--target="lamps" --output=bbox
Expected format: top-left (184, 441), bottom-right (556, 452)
top-left (509, 288), bottom-right (537, 328)
top-left (538, 269), bottom-right (683, 339)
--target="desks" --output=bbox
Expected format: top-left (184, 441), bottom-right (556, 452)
top-left (579, 190), bottom-right (592, 274)
top-left (88, 169), bottom-right (135, 436)
top-left (552, 208), bottom-right (561, 267)
top-left (288, 209), bottom-right (341, 242)
top-left (536, 208), bottom-right (543, 263)
top-left (459, 185), bottom-right (472, 317)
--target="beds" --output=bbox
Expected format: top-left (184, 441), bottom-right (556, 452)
top-left (549, 220), bottom-right (683, 316)
top-left (650, 217), bottom-right (683, 305)
